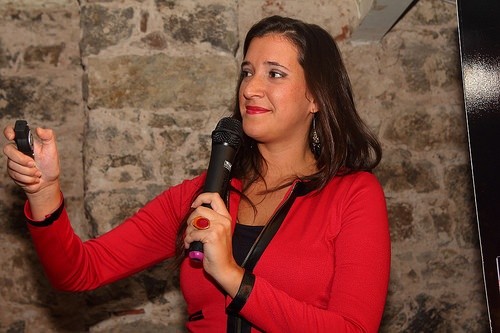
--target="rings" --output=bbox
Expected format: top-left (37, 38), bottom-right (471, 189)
top-left (193, 216), bottom-right (210, 230)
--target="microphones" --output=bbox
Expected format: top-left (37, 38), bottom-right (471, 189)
top-left (189, 117), bottom-right (244, 262)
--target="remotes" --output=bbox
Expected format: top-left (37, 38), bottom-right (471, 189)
top-left (15, 120), bottom-right (34, 162)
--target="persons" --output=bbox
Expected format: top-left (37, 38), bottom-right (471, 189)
top-left (4, 15), bottom-right (390, 333)
